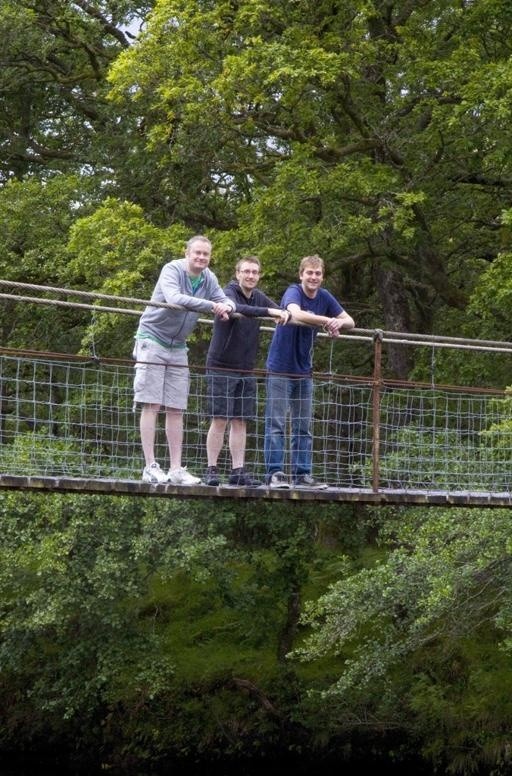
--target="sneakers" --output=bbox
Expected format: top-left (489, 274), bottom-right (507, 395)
top-left (142, 463), bottom-right (171, 483)
top-left (269, 470), bottom-right (290, 489)
top-left (228, 473), bottom-right (262, 488)
top-left (294, 473), bottom-right (328, 490)
top-left (207, 470), bottom-right (219, 485)
top-left (168, 467), bottom-right (201, 485)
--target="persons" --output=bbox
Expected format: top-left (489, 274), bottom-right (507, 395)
top-left (263, 254), bottom-right (355, 489)
top-left (204, 254), bottom-right (292, 489)
top-left (131, 235), bottom-right (236, 484)
